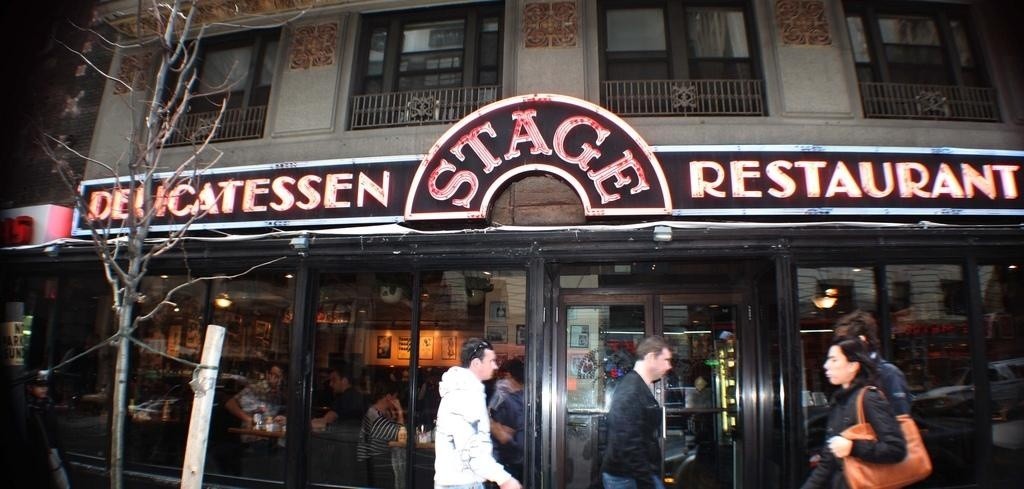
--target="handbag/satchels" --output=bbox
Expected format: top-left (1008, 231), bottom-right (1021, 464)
top-left (839, 415), bottom-right (933, 489)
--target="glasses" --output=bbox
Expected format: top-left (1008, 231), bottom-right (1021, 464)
top-left (470, 341), bottom-right (489, 360)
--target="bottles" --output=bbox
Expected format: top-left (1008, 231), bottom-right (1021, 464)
top-left (265, 417), bottom-right (273, 432)
top-left (398, 426), bottom-right (408, 443)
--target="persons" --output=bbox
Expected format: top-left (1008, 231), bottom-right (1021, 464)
top-left (809, 310), bottom-right (912, 467)
top-left (224, 361), bottom-right (288, 456)
top-left (432, 335), bottom-right (524, 489)
top-left (353, 379), bottom-right (408, 489)
top-left (599, 334), bottom-right (674, 489)
top-left (310, 364), bottom-right (367, 429)
top-left (799, 334), bottom-right (908, 489)
top-left (23, 367), bottom-right (56, 414)
top-left (491, 357), bottom-right (524, 485)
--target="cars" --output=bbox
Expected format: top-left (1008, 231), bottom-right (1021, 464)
top-left (912, 358), bottom-right (1024, 420)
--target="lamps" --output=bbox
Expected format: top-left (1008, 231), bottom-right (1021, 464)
top-left (651, 226), bottom-right (675, 243)
top-left (211, 292), bottom-right (233, 309)
top-left (811, 280), bottom-right (841, 310)
top-left (287, 235), bottom-right (309, 251)
